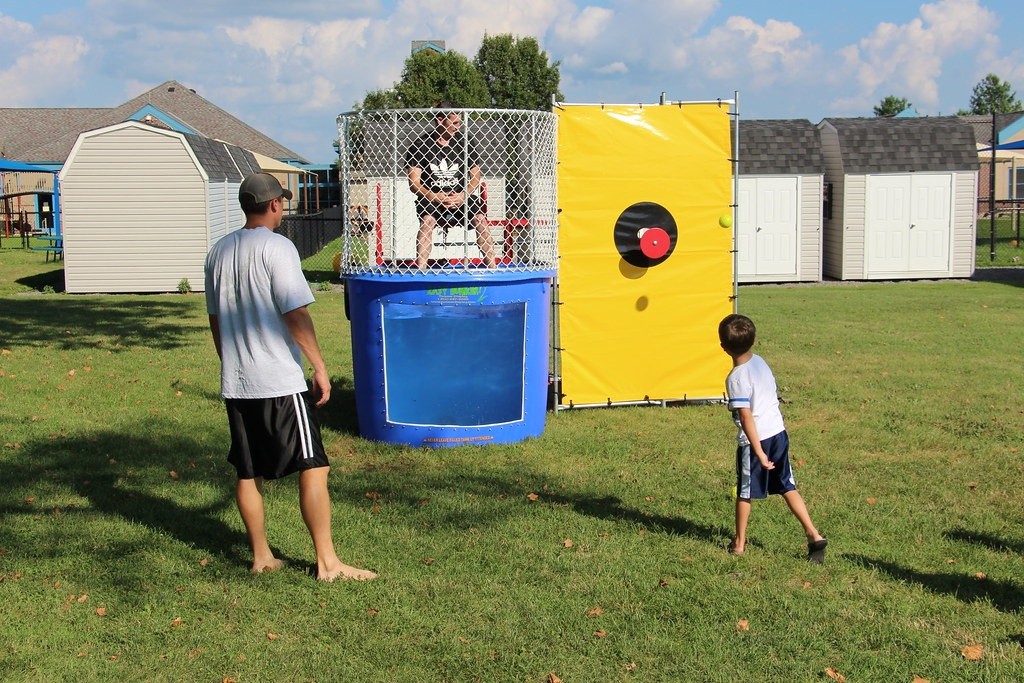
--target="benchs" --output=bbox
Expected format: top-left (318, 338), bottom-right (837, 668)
top-left (31, 247), bottom-right (64, 264)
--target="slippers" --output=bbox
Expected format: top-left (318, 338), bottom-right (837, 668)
top-left (807, 539), bottom-right (827, 566)
top-left (727, 542), bottom-right (744, 556)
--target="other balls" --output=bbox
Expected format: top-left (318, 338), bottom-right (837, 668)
top-left (719, 215), bottom-right (732, 228)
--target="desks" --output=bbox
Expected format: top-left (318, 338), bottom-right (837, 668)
top-left (37, 236), bottom-right (63, 261)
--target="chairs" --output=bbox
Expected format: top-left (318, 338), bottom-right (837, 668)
top-left (434, 182), bottom-right (487, 247)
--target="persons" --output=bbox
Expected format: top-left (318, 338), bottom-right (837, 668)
top-left (403, 101), bottom-right (496, 269)
top-left (204, 173), bottom-right (378, 583)
top-left (350, 206), bottom-right (373, 238)
top-left (719, 314), bottom-right (827, 564)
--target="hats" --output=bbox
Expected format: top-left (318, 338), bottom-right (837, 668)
top-left (237, 171), bottom-right (293, 203)
